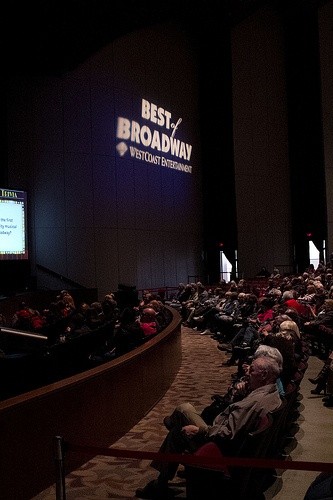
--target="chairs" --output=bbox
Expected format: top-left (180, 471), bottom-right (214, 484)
top-left (0, 273), bottom-right (333, 500)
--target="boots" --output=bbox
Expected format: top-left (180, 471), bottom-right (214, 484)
top-left (309, 366), bottom-right (330, 384)
top-left (310, 379), bottom-right (328, 394)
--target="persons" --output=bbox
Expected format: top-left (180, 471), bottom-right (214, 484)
top-left (0, 288), bottom-right (145, 402)
top-left (134, 356), bottom-right (282, 499)
top-left (136, 308), bottom-right (158, 336)
top-left (165, 259), bottom-right (333, 321)
top-left (200, 344), bottom-right (286, 427)
top-left (181, 315), bottom-right (333, 402)
top-left (131, 289), bottom-right (164, 313)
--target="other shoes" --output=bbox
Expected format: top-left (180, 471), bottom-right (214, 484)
top-left (177, 469), bottom-right (187, 478)
top-left (163, 413), bottom-right (179, 431)
top-left (136, 479), bottom-right (169, 499)
top-left (182, 316), bottom-right (238, 366)
